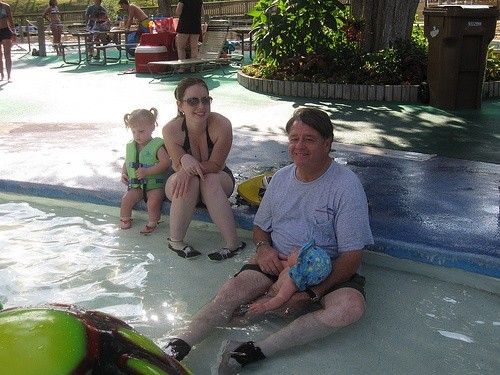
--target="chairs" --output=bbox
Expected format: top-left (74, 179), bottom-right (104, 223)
top-left (148, 20), bottom-right (244, 85)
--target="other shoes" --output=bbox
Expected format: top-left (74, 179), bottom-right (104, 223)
top-left (120, 219), bottom-right (132, 230)
top-left (140, 223), bottom-right (158, 234)
top-left (211, 338), bottom-right (246, 375)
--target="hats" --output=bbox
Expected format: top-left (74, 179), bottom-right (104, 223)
top-left (288, 239), bottom-right (332, 291)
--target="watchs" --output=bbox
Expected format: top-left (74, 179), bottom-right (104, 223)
top-left (306, 288), bottom-right (319, 303)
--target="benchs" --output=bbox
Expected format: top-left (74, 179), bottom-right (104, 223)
top-left (56, 40), bottom-right (125, 64)
top-left (94, 43), bottom-right (139, 66)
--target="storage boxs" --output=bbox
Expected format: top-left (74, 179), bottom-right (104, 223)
top-left (134, 46), bottom-right (168, 73)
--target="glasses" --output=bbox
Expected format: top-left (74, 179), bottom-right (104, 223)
top-left (180, 96), bottom-right (213, 106)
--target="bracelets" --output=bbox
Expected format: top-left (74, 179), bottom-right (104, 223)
top-left (178, 153), bottom-right (184, 164)
top-left (252, 240), bottom-right (270, 252)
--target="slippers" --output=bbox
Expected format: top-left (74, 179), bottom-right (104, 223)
top-left (208, 240), bottom-right (247, 262)
top-left (168, 239), bottom-right (202, 260)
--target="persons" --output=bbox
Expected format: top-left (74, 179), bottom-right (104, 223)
top-left (119, 107), bottom-right (173, 234)
top-left (0, 2), bottom-right (18, 83)
top-left (174, 0), bottom-right (205, 73)
top-left (85, 0), bottom-right (110, 61)
top-left (118, 0), bottom-right (151, 41)
top-left (248, 239), bottom-right (333, 315)
top-left (162, 76), bottom-right (247, 262)
top-left (42, 0), bottom-right (65, 56)
top-left (162, 107), bottom-right (374, 375)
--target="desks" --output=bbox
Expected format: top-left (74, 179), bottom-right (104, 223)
top-left (221, 28), bottom-right (257, 61)
top-left (422, 7), bottom-right (500, 109)
top-left (72, 30), bottom-right (138, 66)
top-left (140, 33), bottom-right (200, 72)
